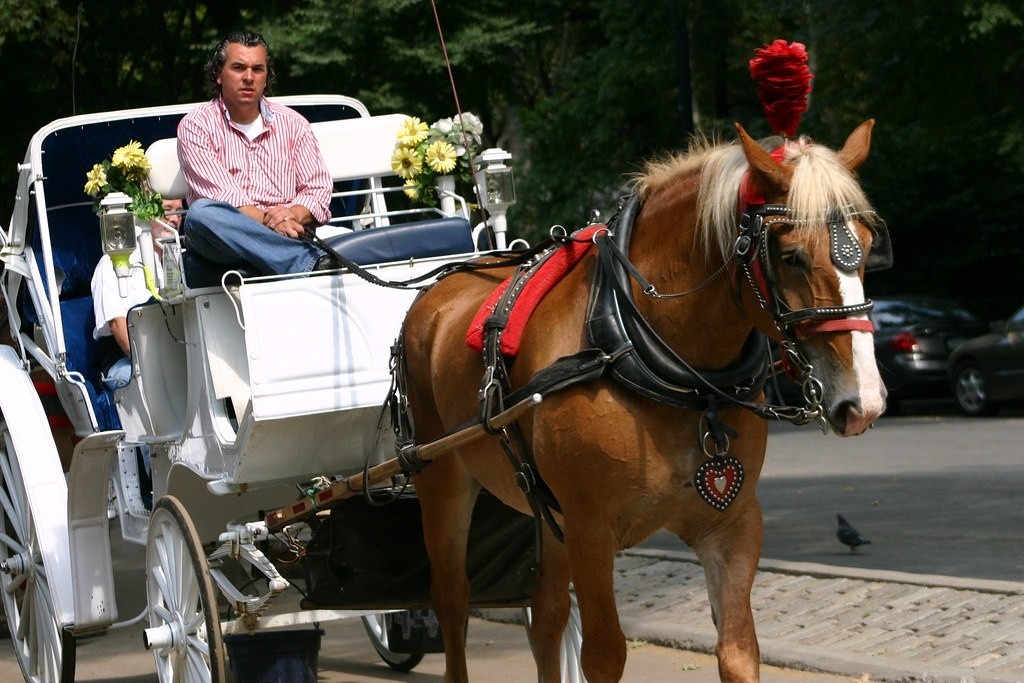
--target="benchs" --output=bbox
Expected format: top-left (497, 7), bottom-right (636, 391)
top-left (29, 202), bottom-right (122, 436)
top-left (145, 114), bottom-right (476, 306)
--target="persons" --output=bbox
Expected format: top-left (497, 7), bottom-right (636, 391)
top-left (90, 196), bottom-right (184, 362)
top-left (175, 30), bottom-right (344, 290)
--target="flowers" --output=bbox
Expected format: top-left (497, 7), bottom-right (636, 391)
top-left (748, 39), bottom-right (814, 138)
top-left (391, 112), bottom-right (483, 206)
top-left (83, 140), bottom-right (171, 222)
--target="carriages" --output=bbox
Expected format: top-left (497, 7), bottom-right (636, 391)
top-left (0, 94), bottom-right (888, 683)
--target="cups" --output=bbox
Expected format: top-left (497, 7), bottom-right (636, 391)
top-left (164, 242), bottom-right (180, 290)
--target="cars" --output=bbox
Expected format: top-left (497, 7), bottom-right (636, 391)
top-left (868, 291), bottom-right (985, 418)
top-left (945, 304), bottom-right (1024, 417)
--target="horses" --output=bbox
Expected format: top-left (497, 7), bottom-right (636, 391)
top-left (396, 119), bottom-right (891, 683)
